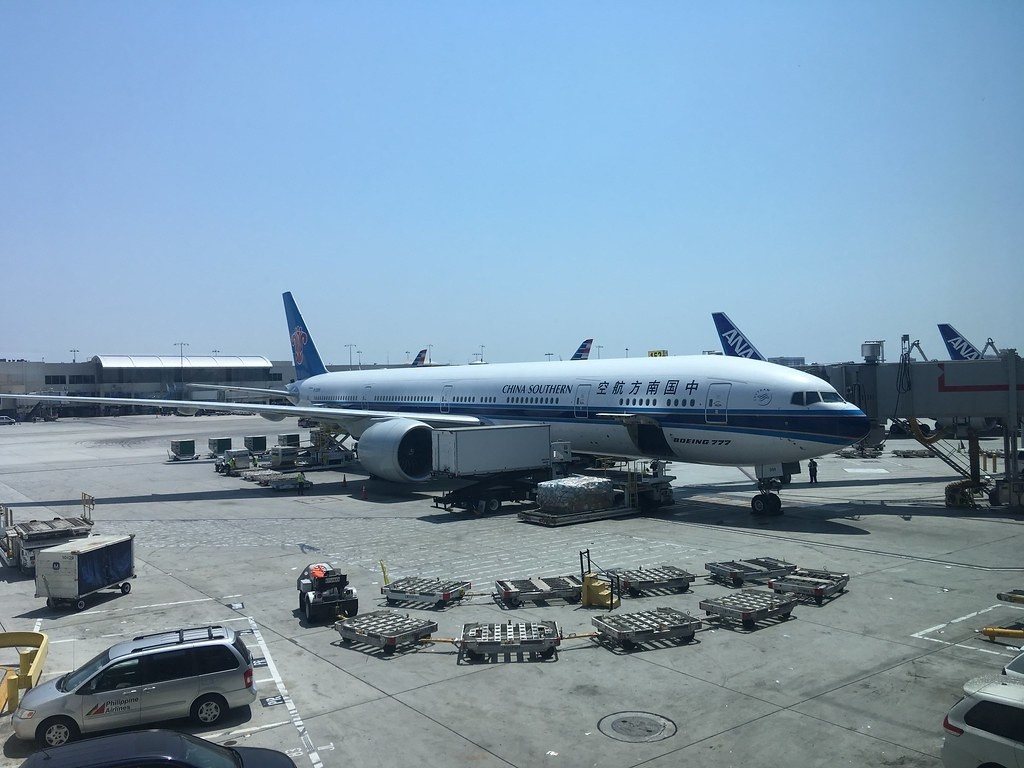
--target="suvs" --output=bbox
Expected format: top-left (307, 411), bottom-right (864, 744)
top-left (10, 624), bottom-right (259, 749)
top-left (19, 727), bottom-right (297, 768)
top-left (941, 643), bottom-right (1024, 768)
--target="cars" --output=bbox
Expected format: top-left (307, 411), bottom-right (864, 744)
top-left (0, 416), bottom-right (16, 425)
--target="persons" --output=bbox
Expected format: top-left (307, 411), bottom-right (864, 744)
top-left (808, 459), bottom-right (818, 484)
top-left (298, 472), bottom-right (305, 495)
top-left (225, 457), bottom-right (235, 475)
top-left (252, 455), bottom-right (258, 467)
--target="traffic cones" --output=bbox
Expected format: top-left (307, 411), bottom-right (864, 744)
top-left (341, 474), bottom-right (349, 488)
top-left (359, 484), bottom-right (368, 500)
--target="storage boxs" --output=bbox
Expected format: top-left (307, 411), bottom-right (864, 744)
top-left (300, 568), bottom-right (354, 604)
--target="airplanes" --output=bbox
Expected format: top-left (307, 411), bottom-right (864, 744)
top-left (888, 322), bottom-right (1024, 439)
top-left (0, 286), bottom-right (874, 525)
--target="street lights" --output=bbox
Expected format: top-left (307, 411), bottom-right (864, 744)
top-left (472, 352), bottom-right (482, 361)
top-left (596, 345), bottom-right (603, 359)
top-left (345, 344), bottom-right (357, 371)
top-left (427, 344), bottom-right (434, 364)
top-left (625, 348), bottom-right (629, 358)
top-left (173, 342), bottom-right (191, 401)
top-left (545, 352), bottom-right (553, 361)
top-left (212, 350), bottom-right (220, 356)
top-left (69, 348), bottom-right (80, 363)
top-left (405, 351), bottom-right (410, 363)
top-left (357, 350), bottom-right (362, 370)
